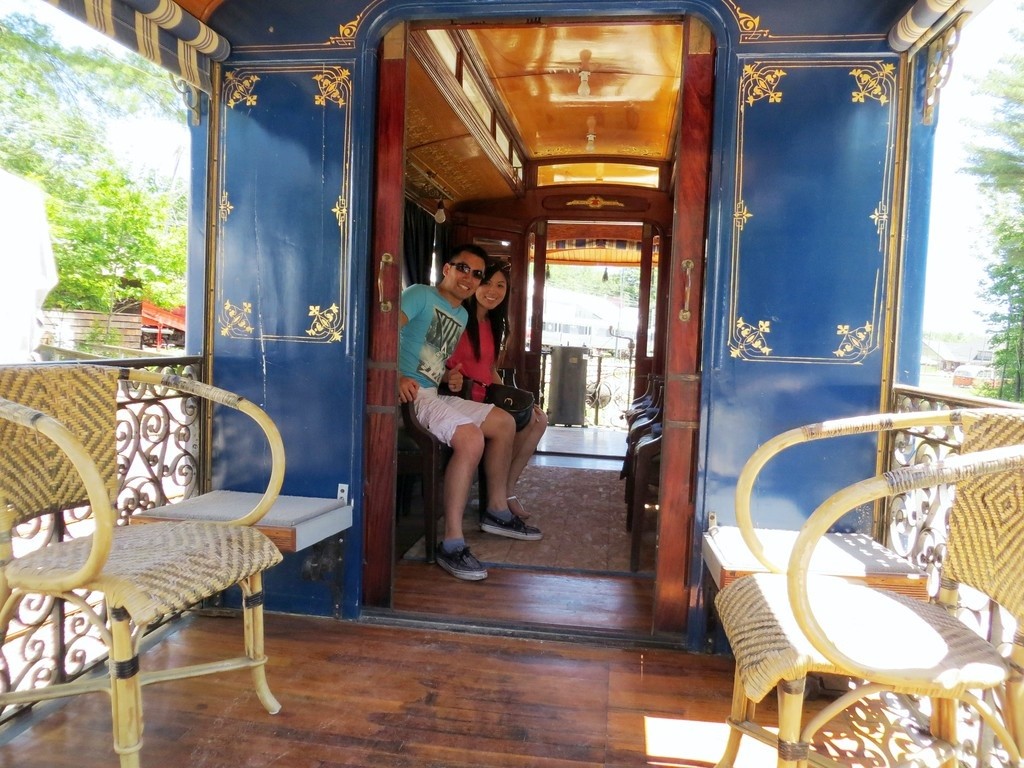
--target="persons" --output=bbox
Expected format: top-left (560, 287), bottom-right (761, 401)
top-left (399, 244), bottom-right (542, 581)
top-left (456, 258), bottom-right (548, 522)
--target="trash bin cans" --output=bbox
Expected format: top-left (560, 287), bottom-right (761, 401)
top-left (547, 346), bottom-right (590, 427)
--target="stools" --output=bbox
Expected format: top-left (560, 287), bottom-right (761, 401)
top-left (700, 527), bottom-right (931, 599)
top-left (124, 488), bottom-right (356, 554)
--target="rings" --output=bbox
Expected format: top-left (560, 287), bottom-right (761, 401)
top-left (453, 385), bottom-right (456, 390)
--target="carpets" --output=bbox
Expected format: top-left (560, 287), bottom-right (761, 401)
top-left (401, 464), bottom-right (657, 577)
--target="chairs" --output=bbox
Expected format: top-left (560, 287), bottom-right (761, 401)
top-left (0, 366), bottom-right (285, 768)
top-left (397, 368), bottom-right (519, 565)
top-left (715, 406), bottom-right (1024, 768)
top-left (618, 373), bottom-right (664, 574)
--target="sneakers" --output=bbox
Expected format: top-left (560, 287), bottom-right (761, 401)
top-left (435, 540), bottom-right (488, 581)
top-left (479, 510), bottom-right (543, 541)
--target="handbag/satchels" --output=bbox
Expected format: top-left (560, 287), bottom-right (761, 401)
top-left (483, 381), bottom-right (535, 432)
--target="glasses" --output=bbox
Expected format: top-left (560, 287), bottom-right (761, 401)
top-left (486, 259), bottom-right (512, 275)
top-left (449, 261), bottom-right (486, 282)
